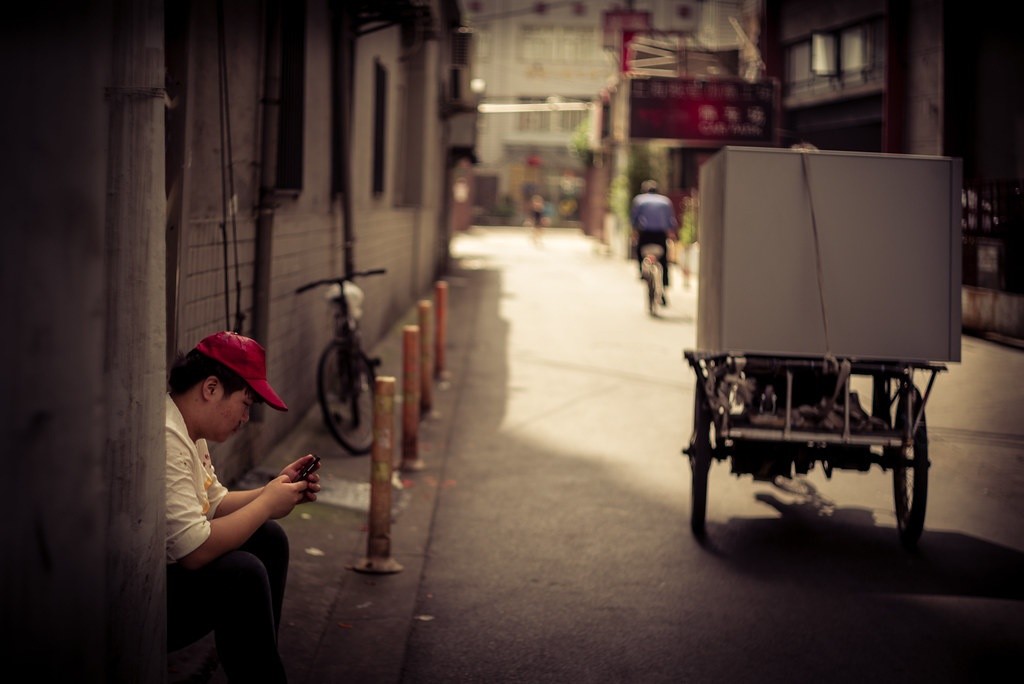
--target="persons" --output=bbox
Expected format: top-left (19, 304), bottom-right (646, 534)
top-left (530, 194), bottom-right (547, 244)
top-left (166, 331), bottom-right (319, 684)
top-left (630, 179), bottom-right (679, 305)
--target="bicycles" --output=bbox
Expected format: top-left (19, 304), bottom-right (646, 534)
top-left (294, 266), bottom-right (383, 457)
top-left (638, 243), bottom-right (664, 317)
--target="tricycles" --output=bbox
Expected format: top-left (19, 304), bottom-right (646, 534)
top-left (681, 349), bottom-right (949, 552)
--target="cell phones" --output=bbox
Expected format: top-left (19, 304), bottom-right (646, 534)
top-left (292, 455), bottom-right (320, 483)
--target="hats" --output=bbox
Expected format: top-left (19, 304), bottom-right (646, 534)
top-left (194, 331), bottom-right (289, 412)
